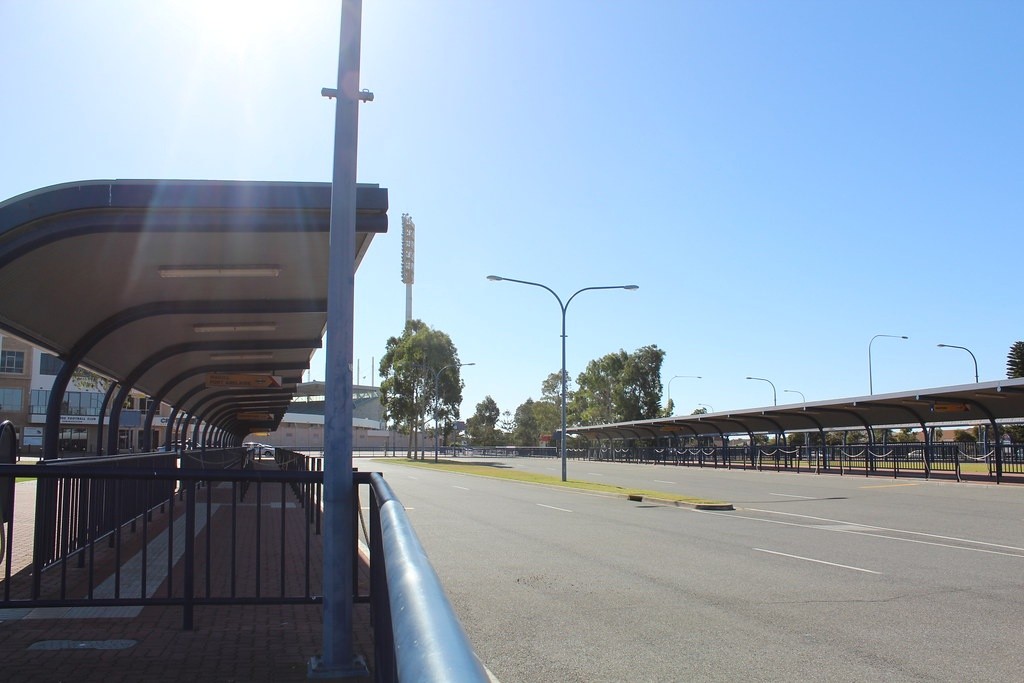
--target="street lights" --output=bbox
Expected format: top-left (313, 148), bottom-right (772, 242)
top-left (397, 363), bottom-right (475, 462)
top-left (668, 375), bottom-right (702, 414)
top-left (938, 344), bottom-right (978, 383)
top-left (486, 276), bottom-right (639, 482)
top-left (784, 390), bottom-right (805, 402)
top-left (746, 377), bottom-right (776, 407)
top-left (868, 335), bottom-right (909, 395)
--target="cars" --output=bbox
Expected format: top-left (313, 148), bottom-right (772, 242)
top-left (157, 440), bottom-right (200, 452)
top-left (243, 442), bottom-right (275, 457)
